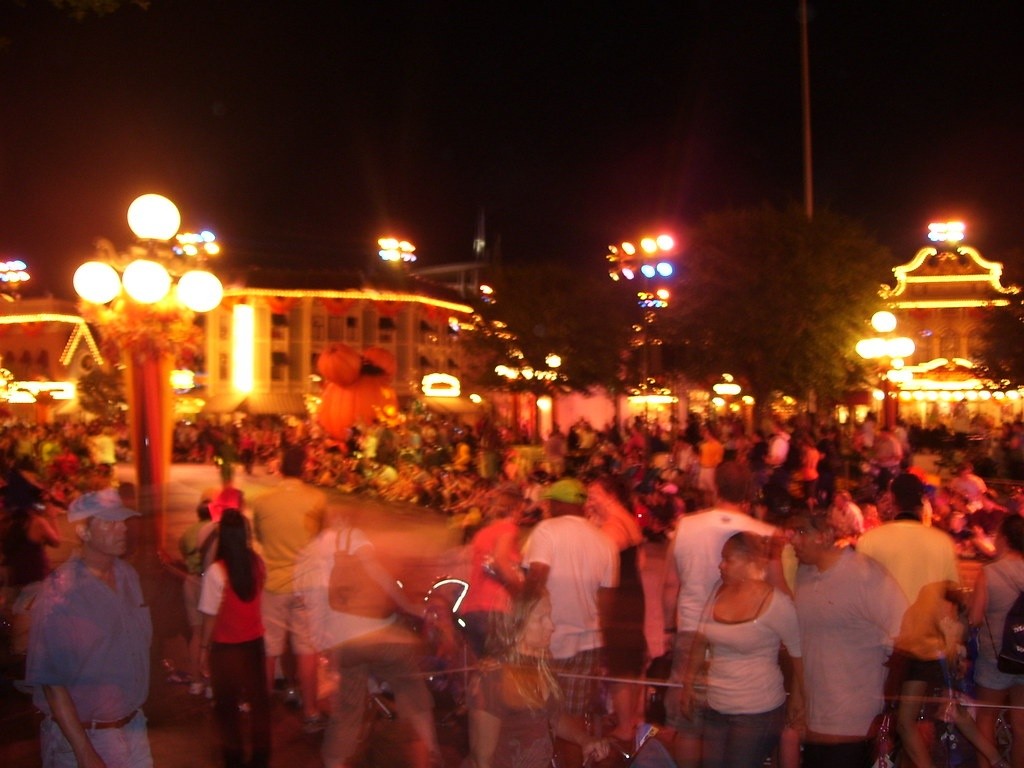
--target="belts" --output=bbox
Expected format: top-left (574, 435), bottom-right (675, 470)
top-left (51, 709), bottom-right (138, 729)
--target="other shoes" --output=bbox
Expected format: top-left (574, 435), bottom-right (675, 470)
top-left (286, 698), bottom-right (298, 707)
top-left (225, 761), bottom-right (268, 768)
top-left (274, 678), bottom-right (284, 689)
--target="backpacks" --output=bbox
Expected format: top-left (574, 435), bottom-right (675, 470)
top-left (985, 562), bottom-right (1024, 675)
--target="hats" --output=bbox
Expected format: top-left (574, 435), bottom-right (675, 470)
top-left (544, 480), bottom-right (588, 504)
top-left (67, 489), bottom-right (142, 522)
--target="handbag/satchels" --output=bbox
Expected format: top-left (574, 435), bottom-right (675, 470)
top-left (329, 527), bottom-right (398, 617)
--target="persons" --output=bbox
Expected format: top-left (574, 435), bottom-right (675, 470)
top-left (0, 397), bottom-right (152, 767)
top-left (171, 404), bottom-right (1024, 768)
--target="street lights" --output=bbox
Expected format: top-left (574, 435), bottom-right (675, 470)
top-left (70, 190), bottom-right (224, 586)
top-left (610, 232), bottom-right (676, 397)
top-left (855, 309), bottom-right (916, 431)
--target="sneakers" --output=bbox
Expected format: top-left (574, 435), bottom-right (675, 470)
top-left (304, 711), bottom-right (335, 733)
